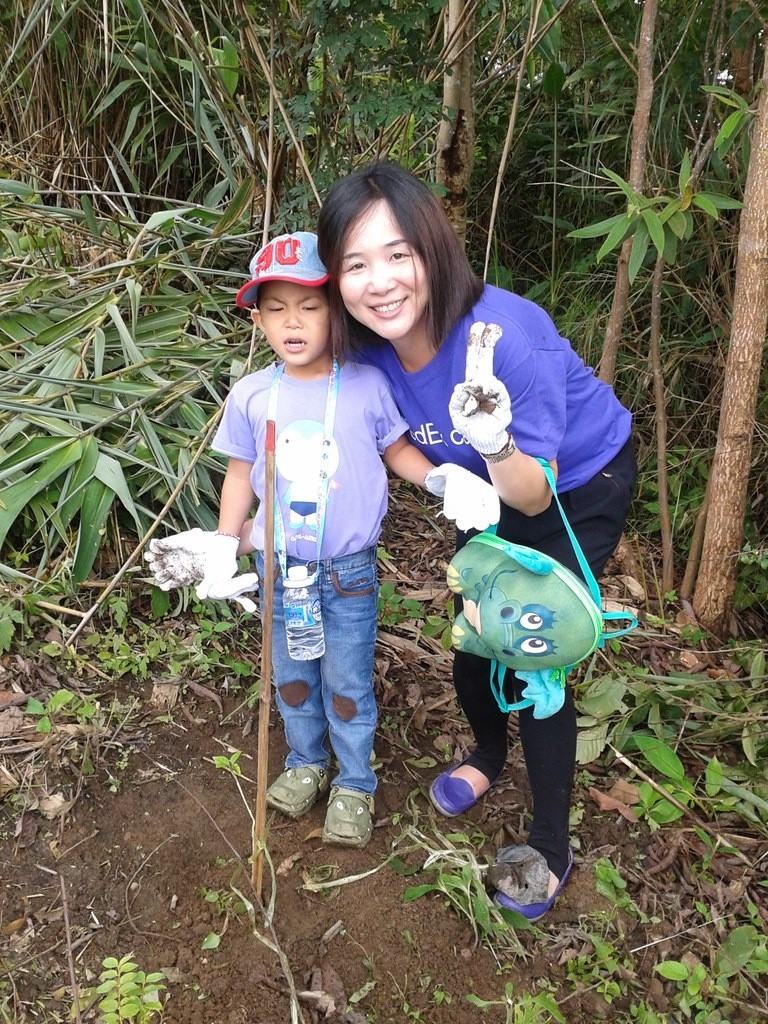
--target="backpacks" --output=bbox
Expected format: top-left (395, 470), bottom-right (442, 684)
top-left (447, 457), bottom-right (638, 720)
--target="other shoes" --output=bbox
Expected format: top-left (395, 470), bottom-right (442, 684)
top-left (265, 764), bottom-right (328, 817)
top-left (321, 786), bottom-right (374, 848)
top-left (493, 842), bottom-right (574, 924)
top-left (429, 756), bottom-right (505, 819)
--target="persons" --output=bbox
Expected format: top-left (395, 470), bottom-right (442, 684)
top-left (141, 155), bottom-right (637, 923)
top-left (196, 230), bottom-right (501, 855)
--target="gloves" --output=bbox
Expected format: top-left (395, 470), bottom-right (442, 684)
top-left (196, 533), bottom-right (257, 613)
top-left (143, 529), bottom-right (210, 591)
top-left (426, 462), bottom-right (499, 532)
top-left (449, 321), bottom-right (513, 455)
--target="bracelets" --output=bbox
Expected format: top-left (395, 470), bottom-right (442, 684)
top-left (483, 433), bottom-right (517, 464)
top-left (218, 532), bottom-right (241, 541)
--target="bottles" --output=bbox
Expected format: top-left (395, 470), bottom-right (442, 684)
top-left (282, 566), bottom-right (325, 660)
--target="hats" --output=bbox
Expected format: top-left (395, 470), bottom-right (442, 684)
top-left (237, 232), bottom-right (328, 305)
